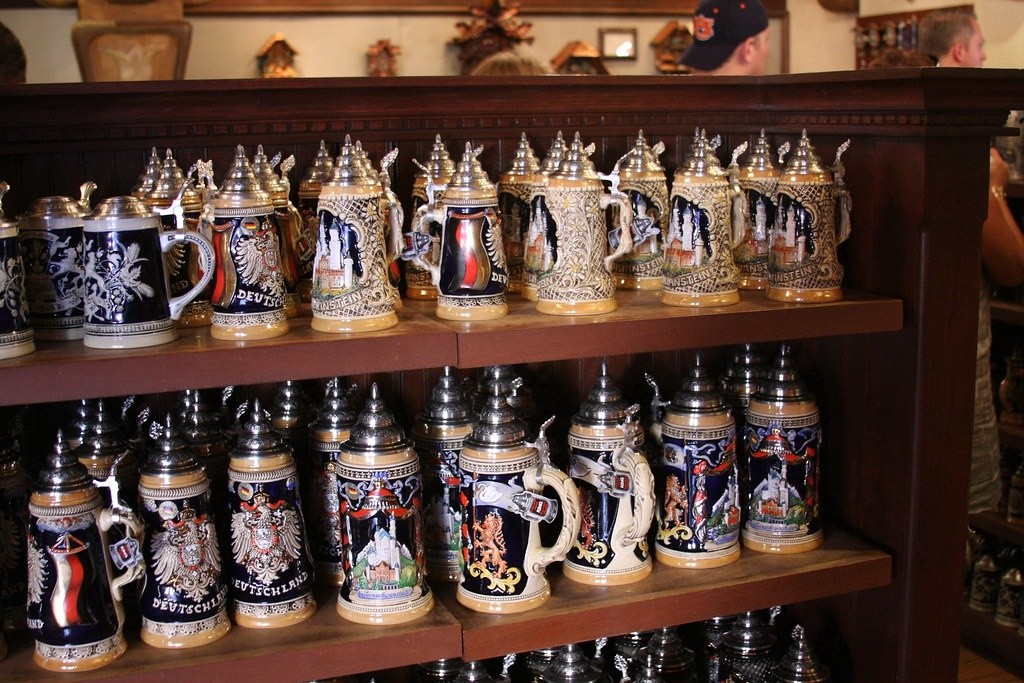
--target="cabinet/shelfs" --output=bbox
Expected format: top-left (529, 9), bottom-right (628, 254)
top-left (0, 65), bottom-right (1024, 683)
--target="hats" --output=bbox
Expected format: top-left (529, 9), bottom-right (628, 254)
top-left (676, 0), bottom-right (769, 70)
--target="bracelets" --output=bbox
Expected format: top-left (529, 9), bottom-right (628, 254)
top-left (991, 185), bottom-right (1006, 199)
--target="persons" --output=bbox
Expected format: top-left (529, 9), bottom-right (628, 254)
top-left (868, 50), bottom-right (1024, 515)
top-left (675, 0), bottom-right (769, 74)
top-left (918, 11), bottom-right (986, 67)
top-left (471, 51), bottom-right (557, 76)
